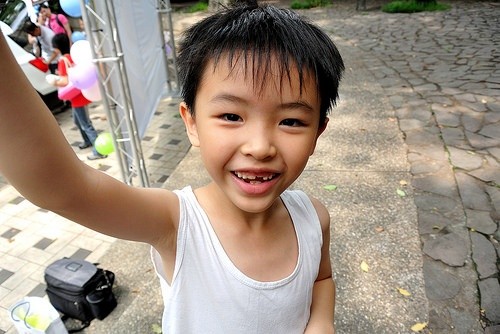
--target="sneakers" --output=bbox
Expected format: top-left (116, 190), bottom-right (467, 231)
top-left (86, 154), bottom-right (108, 160)
top-left (78, 142), bottom-right (92, 149)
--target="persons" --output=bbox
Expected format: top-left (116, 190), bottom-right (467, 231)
top-left (23, 22), bottom-right (61, 74)
top-left (0, 4), bottom-right (345, 334)
top-left (35, 4), bottom-right (72, 48)
top-left (46, 33), bottom-right (108, 160)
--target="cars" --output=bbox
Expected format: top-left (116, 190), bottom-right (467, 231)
top-left (1, 0), bottom-right (67, 115)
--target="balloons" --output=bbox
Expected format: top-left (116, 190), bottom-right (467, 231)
top-left (67, 31), bottom-right (102, 103)
top-left (95, 132), bottom-right (115, 156)
top-left (60, 0), bottom-right (90, 17)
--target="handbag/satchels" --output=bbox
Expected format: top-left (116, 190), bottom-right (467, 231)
top-left (43, 257), bottom-right (118, 334)
top-left (58, 56), bottom-right (81, 100)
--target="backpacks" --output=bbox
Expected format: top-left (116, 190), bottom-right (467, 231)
top-left (55, 13), bottom-right (74, 33)
